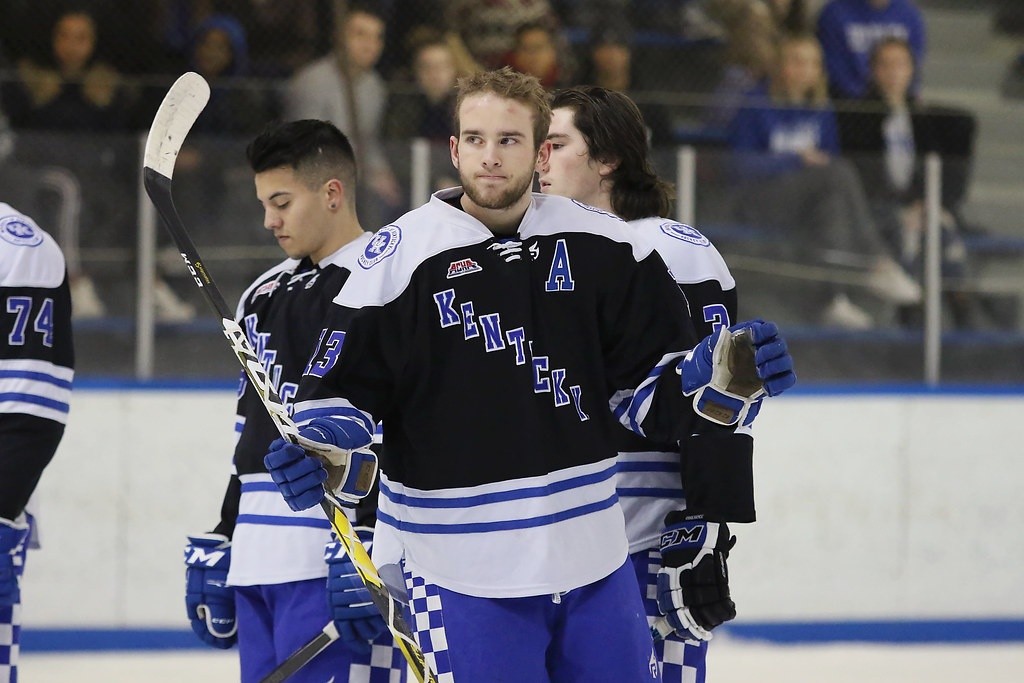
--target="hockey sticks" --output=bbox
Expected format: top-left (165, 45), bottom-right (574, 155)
top-left (263, 613), bottom-right (340, 683)
top-left (143, 68), bottom-right (438, 682)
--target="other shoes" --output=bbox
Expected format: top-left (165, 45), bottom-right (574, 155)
top-left (870, 265), bottom-right (920, 304)
top-left (818, 297), bottom-right (874, 330)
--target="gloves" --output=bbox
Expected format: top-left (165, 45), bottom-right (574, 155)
top-left (675, 320), bottom-right (798, 429)
top-left (184, 534), bottom-right (238, 650)
top-left (0, 510), bottom-right (40, 609)
top-left (263, 415), bottom-right (377, 513)
top-left (323, 526), bottom-right (393, 656)
top-left (657, 511), bottom-right (736, 641)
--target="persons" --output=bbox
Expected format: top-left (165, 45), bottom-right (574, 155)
top-left (540, 84), bottom-right (739, 683)
top-left (384, 40), bottom-right (460, 190)
top-left (264, 68), bottom-right (796, 683)
top-left (844, 37), bottom-right (967, 265)
top-left (0, 199), bottom-right (75, 683)
top-left (445, 0), bottom-right (822, 108)
top-left (183, 119), bottom-right (382, 683)
top-left (726, 37), bottom-right (921, 328)
top-left (574, 42), bottom-right (669, 145)
top-left (483, 20), bottom-right (561, 93)
top-left (1, 0), bottom-right (401, 209)
top-left (817, 0), bottom-right (925, 97)
